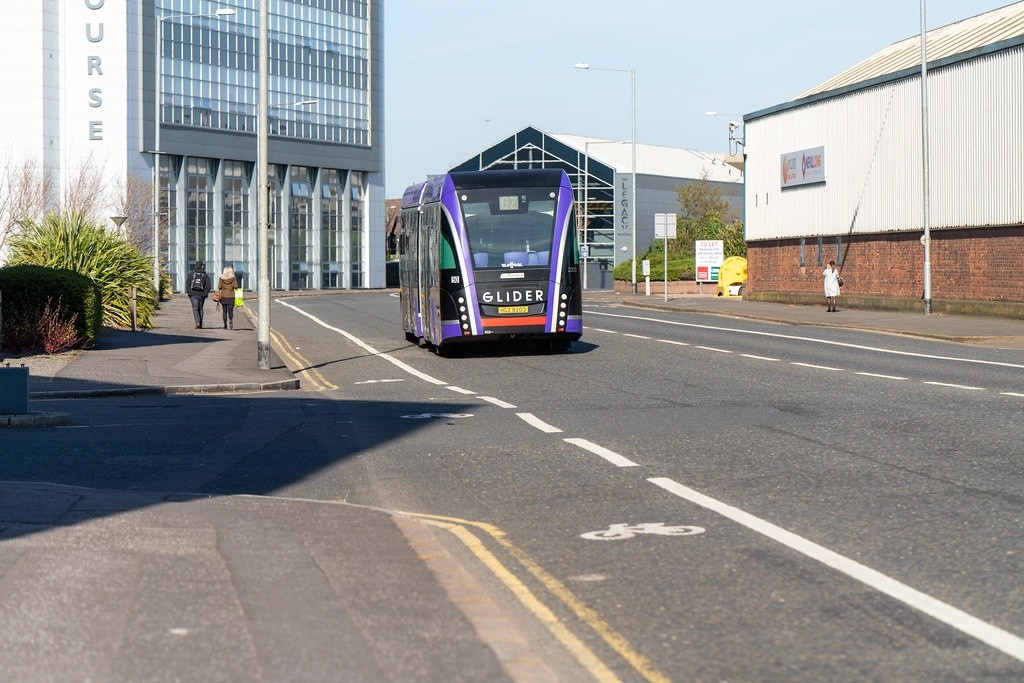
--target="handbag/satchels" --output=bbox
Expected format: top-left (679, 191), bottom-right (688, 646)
top-left (212, 289), bottom-right (222, 302)
top-left (837, 277), bottom-right (843, 287)
top-left (233, 288), bottom-right (244, 307)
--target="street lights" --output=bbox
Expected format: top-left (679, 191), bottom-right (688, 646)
top-left (572, 63), bottom-right (638, 295)
top-left (582, 139), bottom-right (634, 292)
top-left (153, 8), bottom-right (237, 303)
top-left (256, 100), bottom-right (319, 213)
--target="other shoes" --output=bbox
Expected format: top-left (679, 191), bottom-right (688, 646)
top-left (224, 324), bottom-right (227, 329)
top-left (826, 308), bottom-right (830, 312)
top-left (229, 322), bottom-right (233, 329)
top-left (199, 321), bottom-right (202, 326)
top-left (195, 323), bottom-right (201, 329)
top-left (831, 307), bottom-right (835, 311)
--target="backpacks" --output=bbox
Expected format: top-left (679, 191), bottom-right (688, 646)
top-left (191, 270), bottom-right (206, 292)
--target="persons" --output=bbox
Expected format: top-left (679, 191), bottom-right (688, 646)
top-left (217, 264), bottom-right (239, 330)
top-left (186, 261), bottom-right (211, 328)
top-left (822, 261), bottom-right (840, 312)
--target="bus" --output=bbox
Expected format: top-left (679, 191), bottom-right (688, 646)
top-left (396, 168), bottom-right (583, 357)
